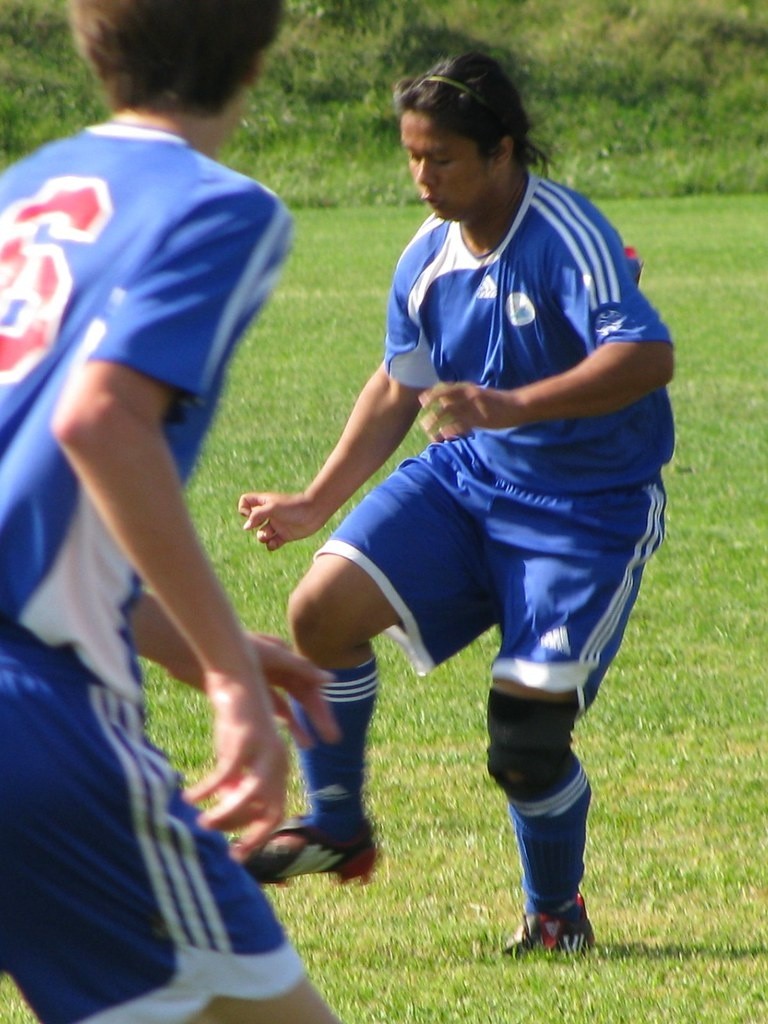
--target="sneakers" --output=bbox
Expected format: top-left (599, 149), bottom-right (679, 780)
top-left (501, 891), bottom-right (596, 961)
top-left (221, 814), bottom-right (377, 887)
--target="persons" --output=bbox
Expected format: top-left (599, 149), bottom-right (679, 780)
top-left (235, 51), bottom-right (677, 958)
top-left (0, 0), bottom-right (344, 1024)
